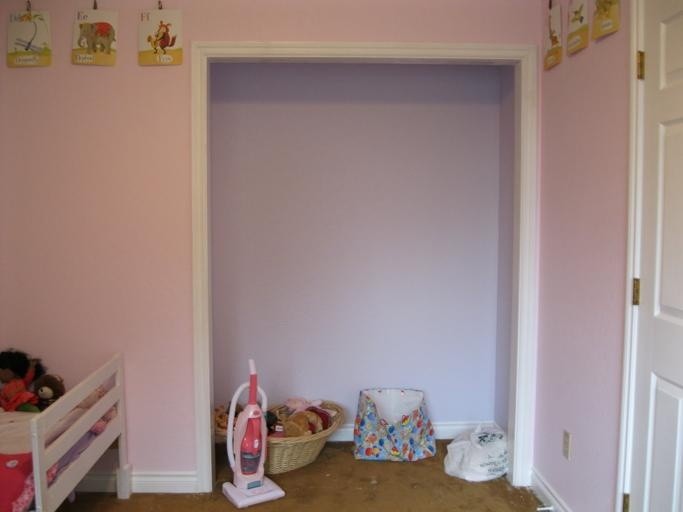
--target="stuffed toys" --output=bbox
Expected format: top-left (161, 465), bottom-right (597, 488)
top-left (0, 348), bottom-right (117, 446)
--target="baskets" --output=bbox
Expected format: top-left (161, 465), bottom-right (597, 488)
top-left (213, 401), bottom-right (346, 475)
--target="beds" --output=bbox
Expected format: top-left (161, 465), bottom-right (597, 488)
top-left (0, 348), bottom-right (134, 512)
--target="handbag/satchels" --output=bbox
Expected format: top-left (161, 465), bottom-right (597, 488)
top-left (352, 388), bottom-right (436, 462)
